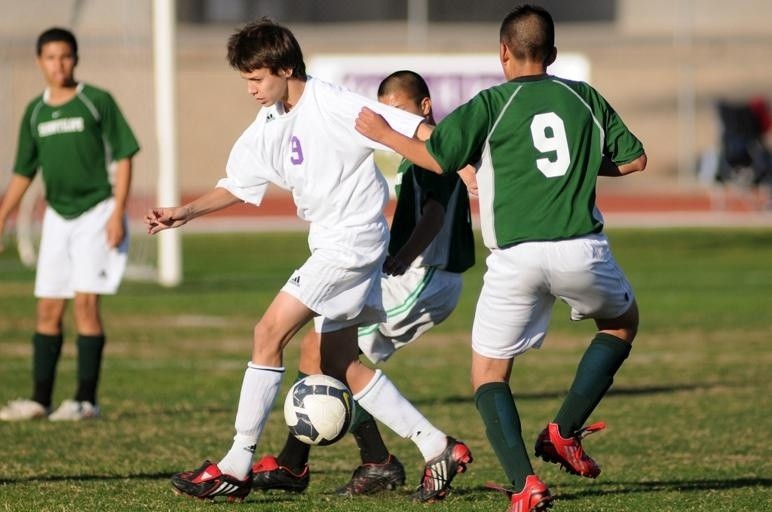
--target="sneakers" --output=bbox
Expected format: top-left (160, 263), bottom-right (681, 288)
top-left (534, 423), bottom-right (608, 477)
top-left (485, 473), bottom-right (553, 512)
top-left (407, 436), bottom-right (473, 504)
top-left (0, 396), bottom-right (47, 422)
top-left (170, 458), bottom-right (250, 503)
top-left (252, 454), bottom-right (309, 493)
top-left (336, 454), bottom-right (406, 496)
top-left (50, 397), bottom-right (98, 422)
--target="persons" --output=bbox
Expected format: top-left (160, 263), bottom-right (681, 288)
top-left (354, 4), bottom-right (647, 512)
top-left (143, 20), bottom-right (481, 502)
top-left (251, 70), bottom-right (475, 497)
top-left (0, 28), bottom-right (140, 424)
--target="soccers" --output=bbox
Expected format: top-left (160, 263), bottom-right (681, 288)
top-left (284, 374), bottom-right (354, 444)
